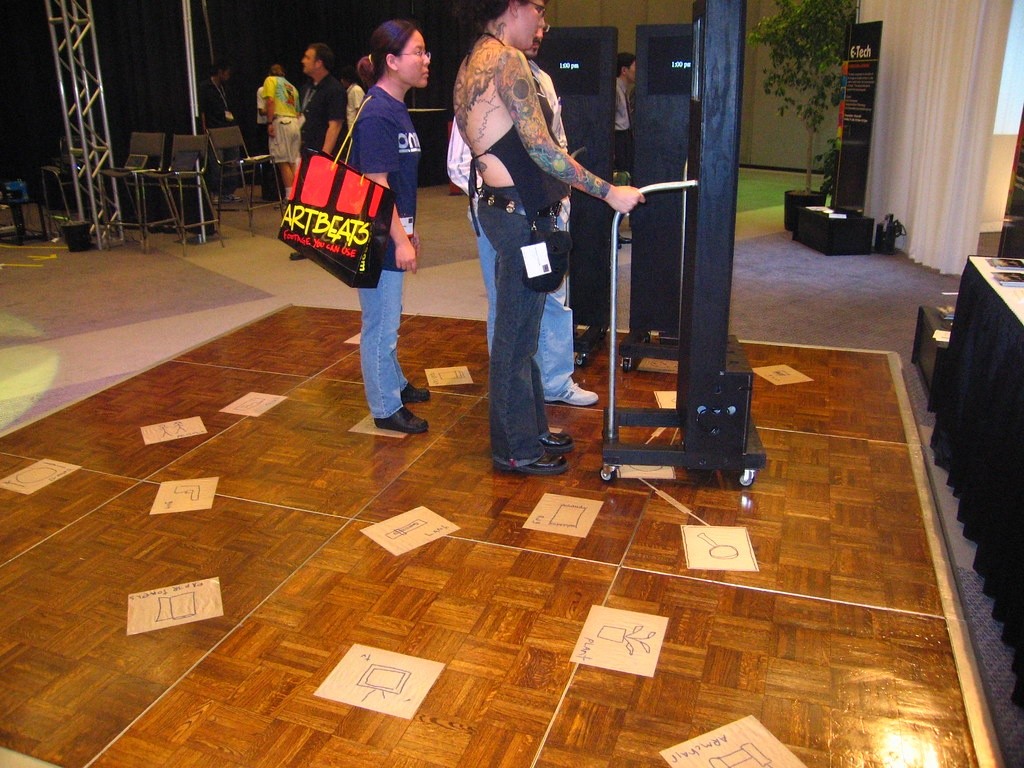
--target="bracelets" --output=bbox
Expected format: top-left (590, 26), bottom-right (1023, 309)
top-left (267, 122), bottom-right (272, 125)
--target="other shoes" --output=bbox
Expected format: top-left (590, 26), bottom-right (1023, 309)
top-left (374, 406), bottom-right (429, 434)
top-left (211, 194), bottom-right (242, 204)
top-left (400, 382), bottom-right (430, 405)
top-left (274, 198), bottom-right (289, 210)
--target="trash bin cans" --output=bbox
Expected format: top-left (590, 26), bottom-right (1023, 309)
top-left (259, 164), bottom-right (286, 201)
top-left (61, 220), bottom-right (92, 252)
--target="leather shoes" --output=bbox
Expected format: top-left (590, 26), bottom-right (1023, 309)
top-left (539, 433), bottom-right (574, 453)
top-left (494, 451), bottom-right (567, 474)
top-left (617, 235), bottom-right (631, 249)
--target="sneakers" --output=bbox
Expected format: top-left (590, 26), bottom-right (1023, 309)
top-left (544, 382), bottom-right (598, 405)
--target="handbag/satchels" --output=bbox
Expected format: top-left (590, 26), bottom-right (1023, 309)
top-left (278, 148), bottom-right (397, 289)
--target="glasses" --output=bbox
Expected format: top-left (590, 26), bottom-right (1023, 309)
top-left (530, 2), bottom-right (546, 16)
top-left (538, 23), bottom-right (551, 32)
top-left (396, 51), bottom-right (431, 61)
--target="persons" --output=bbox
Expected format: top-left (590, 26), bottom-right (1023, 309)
top-left (198, 42), bottom-right (376, 261)
top-left (447, 0), bottom-right (646, 476)
top-left (345, 19), bottom-right (431, 434)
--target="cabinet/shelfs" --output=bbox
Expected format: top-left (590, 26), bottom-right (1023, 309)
top-left (909, 304), bottom-right (955, 414)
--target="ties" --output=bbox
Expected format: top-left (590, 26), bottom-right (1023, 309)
top-left (624, 92), bottom-right (633, 132)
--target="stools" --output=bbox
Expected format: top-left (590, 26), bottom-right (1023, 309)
top-left (99, 131), bottom-right (183, 248)
top-left (138, 133), bottom-right (226, 257)
top-left (41, 128), bottom-right (100, 221)
top-left (206, 125), bottom-right (295, 238)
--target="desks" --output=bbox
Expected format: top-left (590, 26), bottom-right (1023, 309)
top-left (934, 257), bottom-right (1024, 703)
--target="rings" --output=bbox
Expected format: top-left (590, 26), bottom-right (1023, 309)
top-left (401, 264), bottom-right (406, 267)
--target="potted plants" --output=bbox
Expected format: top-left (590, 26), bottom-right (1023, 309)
top-left (744, 0), bottom-right (856, 233)
